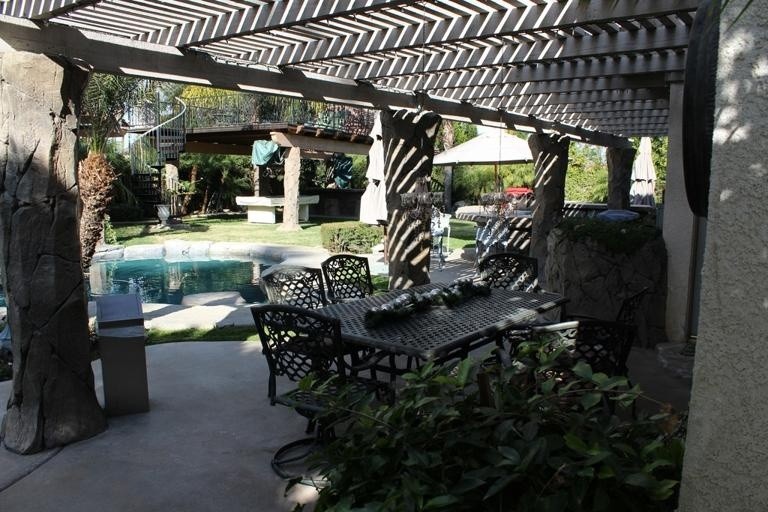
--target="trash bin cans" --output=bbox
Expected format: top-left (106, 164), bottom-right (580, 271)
top-left (96, 293), bottom-right (150, 417)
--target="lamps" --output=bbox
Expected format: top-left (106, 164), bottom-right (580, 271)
top-left (400, 0), bottom-right (447, 253)
top-left (478, 107), bottom-right (516, 233)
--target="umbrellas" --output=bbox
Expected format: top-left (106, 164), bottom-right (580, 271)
top-left (628, 135), bottom-right (657, 208)
top-left (432, 123), bottom-right (572, 213)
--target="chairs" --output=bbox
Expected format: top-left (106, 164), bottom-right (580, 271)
top-left (251, 304), bottom-right (388, 439)
top-left (261, 254), bottom-right (383, 404)
top-left (480, 254), bottom-right (544, 358)
top-left (429, 207), bottom-right (446, 272)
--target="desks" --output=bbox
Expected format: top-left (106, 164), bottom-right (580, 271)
top-left (264, 282), bottom-right (569, 385)
top-left (236, 194), bottom-right (319, 224)
